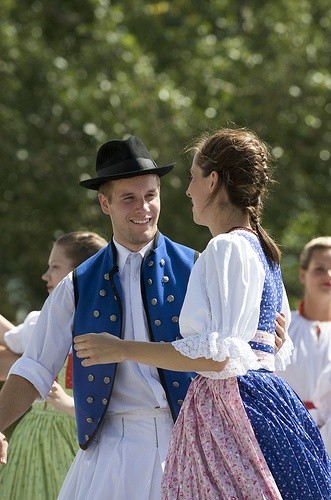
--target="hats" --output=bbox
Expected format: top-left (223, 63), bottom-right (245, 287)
top-left (79, 136), bottom-right (175, 190)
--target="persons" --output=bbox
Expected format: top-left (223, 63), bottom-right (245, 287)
top-left (274, 236), bottom-right (331, 458)
top-left (0, 136), bottom-right (286, 500)
top-left (73, 129), bottom-right (331, 500)
top-left (0, 232), bottom-right (107, 500)
